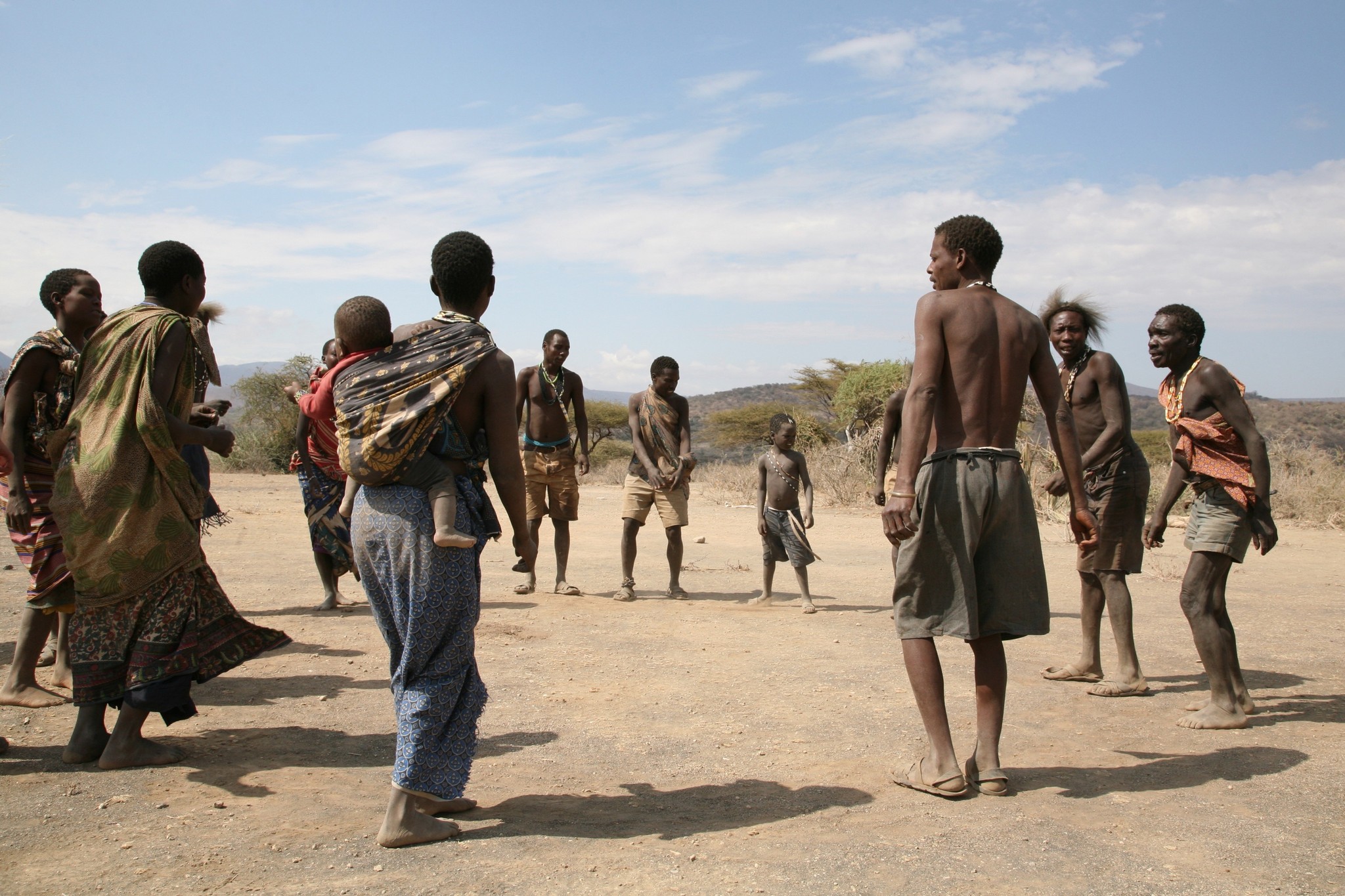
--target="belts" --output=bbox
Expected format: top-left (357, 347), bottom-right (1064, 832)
top-left (519, 439), bottom-right (571, 453)
top-left (1193, 478), bottom-right (1219, 494)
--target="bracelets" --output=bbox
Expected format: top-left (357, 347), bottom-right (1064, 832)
top-left (293, 389), bottom-right (308, 403)
top-left (308, 476), bottom-right (318, 484)
top-left (889, 491), bottom-right (918, 498)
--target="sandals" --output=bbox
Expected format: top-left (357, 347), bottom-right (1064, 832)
top-left (747, 597), bottom-right (772, 608)
top-left (801, 603), bottom-right (815, 614)
top-left (1039, 664), bottom-right (1105, 682)
top-left (665, 586), bottom-right (689, 600)
top-left (613, 587), bottom-right (637, 601)
top-left (1086, 676), bottom-right (1148, 697)
top-left (513, 581), bottom-right (536, 593)
top-left (888, 756), bottom-right (968, 796)
top-left (554, 583), bottom-right (580, 595)
top-left (958, 761), bottom-right (1008, 796)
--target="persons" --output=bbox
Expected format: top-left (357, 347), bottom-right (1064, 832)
top-left (611, 356), bottom-right (695, 600)
top-left (45, 240), bottom-right (294, 769)
top-left (747, 413), bottom-right (820, 614)
top-left (28, 307), bottom-right (110, 667)
top-left (285, 291), bottom-right (481, 552)
top-left (1141, 303), bottom-right (1278, 731)
top-left (1038, 303), bottom-right (1151, 698)
top-left (287, 338), bottom-right (369, 610)
top-left (0, 269), bottom-right (101, 709)
top-left (341, 230), bottom-right (535, 850)
top-left (873, 364), bottom-right (915, 508)
top-left (513, 330), bottom-right (590, 596)
top-left (880, 214), bottom-right (1102, 797)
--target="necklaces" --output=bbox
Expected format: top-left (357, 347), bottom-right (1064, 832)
top-left (965, 281), bottom-right (997, 292)
top-left (1056, 346), bottom-right (1092, 407)
top-left (1163, 356), bottom-right (1204, 423)
top-left (537, 362), bottom-right (566, 406)
